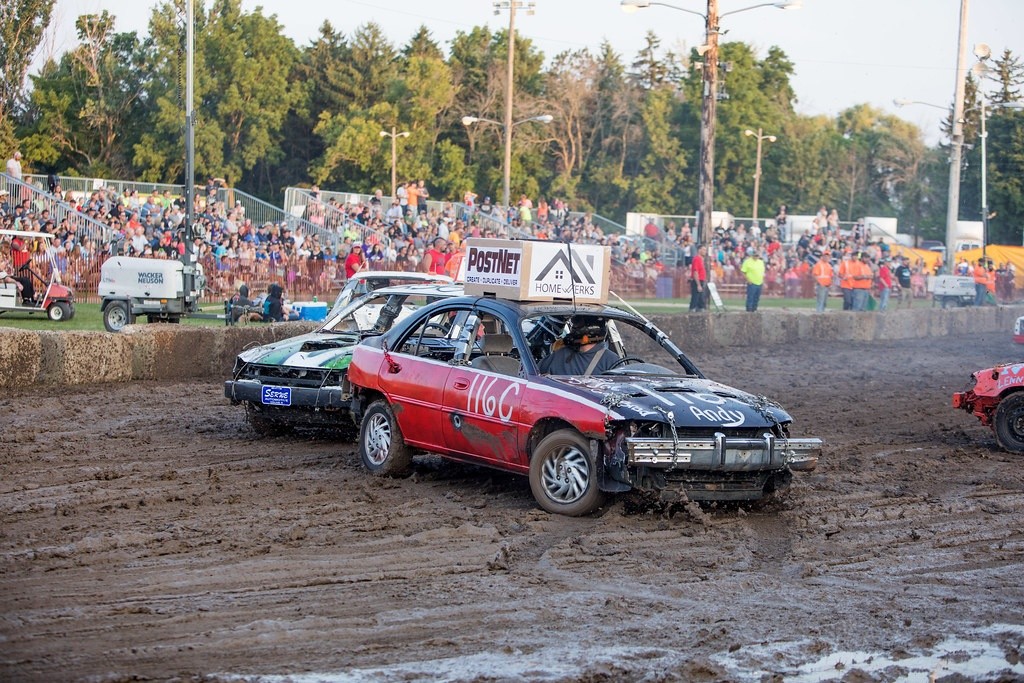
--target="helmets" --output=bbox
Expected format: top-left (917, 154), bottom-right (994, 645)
top-left (563, 315), bottom-right (609, 351)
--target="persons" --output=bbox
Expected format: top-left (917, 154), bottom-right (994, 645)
top-left (537, 315), bottom-right (625, 376)
top-left (0, 151), bottom-right (1017, 323)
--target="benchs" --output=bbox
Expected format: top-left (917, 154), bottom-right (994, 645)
top-left (622, 284), bottom-right (901, 296)
top-left (250, 279), bottom-right (347, 291)
top-left (0, 276), bottom-right (19, 289)
top-left (419, 349), bottom-right (521, 364)
top-left (660, 252), bottom-right (682, 265)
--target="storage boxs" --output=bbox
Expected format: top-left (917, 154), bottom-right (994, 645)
top-left (464, 238), bottom-right (611, 304)
top-left (292, 301), bottom-right (328, 322)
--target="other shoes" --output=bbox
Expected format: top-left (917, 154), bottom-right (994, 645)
top-left (22, 299), bottom-right (35, 307)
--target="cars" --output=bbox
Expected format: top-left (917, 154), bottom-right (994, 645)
top-left (951, 360), bottom-right (1024, 456)
top-left (338, 292), bottom-right (825, 518)
top-left (224, 269), bottom-right (538, 437)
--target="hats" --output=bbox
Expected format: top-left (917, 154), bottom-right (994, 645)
top-left (353, 241), bottom-right (362, 248)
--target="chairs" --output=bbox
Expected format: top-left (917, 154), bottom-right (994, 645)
top-left (471, 333), bottom-right (521, 377)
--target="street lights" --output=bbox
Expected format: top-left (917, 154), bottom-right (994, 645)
top-left (745, 127), bottom-right (777, 240)
top-left (460, 0), bottom-right (554, 236)
top-left (378, 126), bottom-right (411, 208)
top-left (620, 0), bottom-right (805, 316)
top-left (893, 96), bottom-right (1024, 277)
